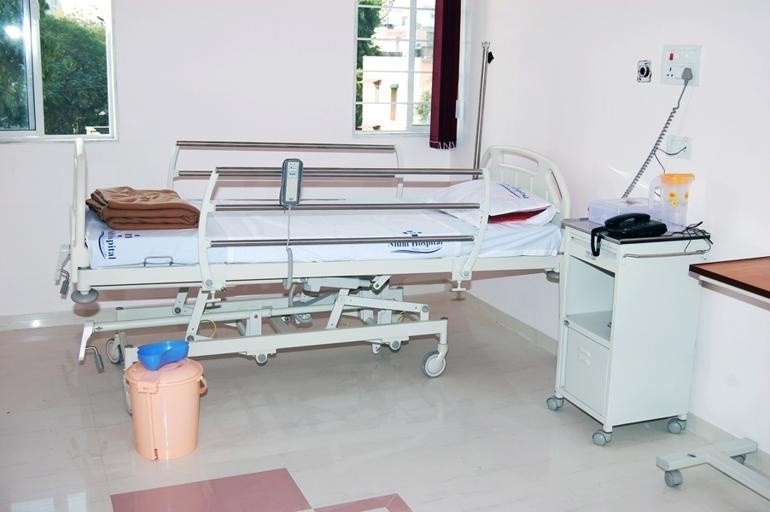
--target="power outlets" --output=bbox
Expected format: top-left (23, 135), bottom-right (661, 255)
top-left (661, 63), bottom-right (698, 87)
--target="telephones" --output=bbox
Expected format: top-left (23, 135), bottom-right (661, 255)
top-left (284, 161), bottom-right (301, 203)
top-left (605, 213), bottom-right (667, 240)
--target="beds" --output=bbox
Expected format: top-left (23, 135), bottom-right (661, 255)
top-left (52, 139), bottom-right (573, 411)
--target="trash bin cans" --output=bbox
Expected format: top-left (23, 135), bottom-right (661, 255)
top-left (123, 358), bottom-right (207, 461)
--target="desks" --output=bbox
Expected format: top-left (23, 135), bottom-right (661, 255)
top-left (654, 254), bottom-right (769, 502)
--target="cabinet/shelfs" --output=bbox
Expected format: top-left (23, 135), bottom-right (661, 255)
top-left (547, 215), bottom-right (712, 447)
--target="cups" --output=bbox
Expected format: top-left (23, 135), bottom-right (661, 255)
top-left (649, 173), bottom-right (694, 229)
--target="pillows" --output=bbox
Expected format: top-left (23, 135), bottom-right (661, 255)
top-left (425, 178), bottom-right (560, 232)
